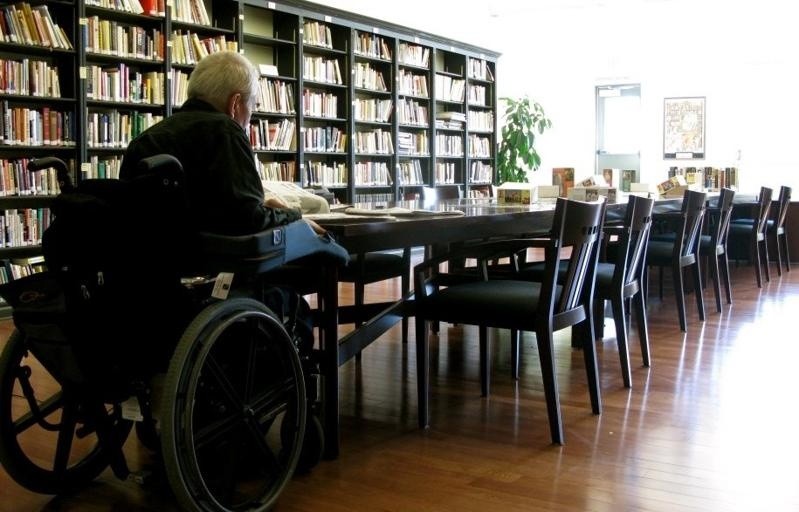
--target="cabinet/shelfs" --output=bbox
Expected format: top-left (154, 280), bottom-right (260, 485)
top-left (0, 1), bottom-right (502, 318)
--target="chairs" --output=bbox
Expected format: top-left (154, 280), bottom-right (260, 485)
top-left (607, 188), bottom-right (707, 332)
top-left (415, 194), bottom-right (609, 445)
top-left (729, 186), bottom-right (792, 276)
top-left (649, 188), bottom-right (735, 313)
top-left (421, 185), bottom-right (526, 333)
top-left (511, 192), bottom-right (656, 388)
top-left (314, 193), bottom-right (410, 364)
top-left (709, 187), bottom-right (773, 288)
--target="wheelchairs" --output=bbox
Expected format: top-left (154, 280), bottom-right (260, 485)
top-left (0, 153), bottom-right (351, 512)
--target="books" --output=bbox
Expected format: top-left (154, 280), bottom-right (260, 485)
top-left (244, 20), bottom-right (495, 221)
top-left (499, 166), bottom-right (736, 205)
top-left (0, 2), bottom-right (237, 307)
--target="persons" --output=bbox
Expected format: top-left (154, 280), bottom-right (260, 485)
top-left (119, 52), bottom-right (324, 480)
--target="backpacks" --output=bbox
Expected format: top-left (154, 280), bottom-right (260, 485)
top-left (0, 180), bottom-right (181, 404)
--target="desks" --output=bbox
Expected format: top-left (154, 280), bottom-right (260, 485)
top-left (302, 195), bottom-right (758, 461)
